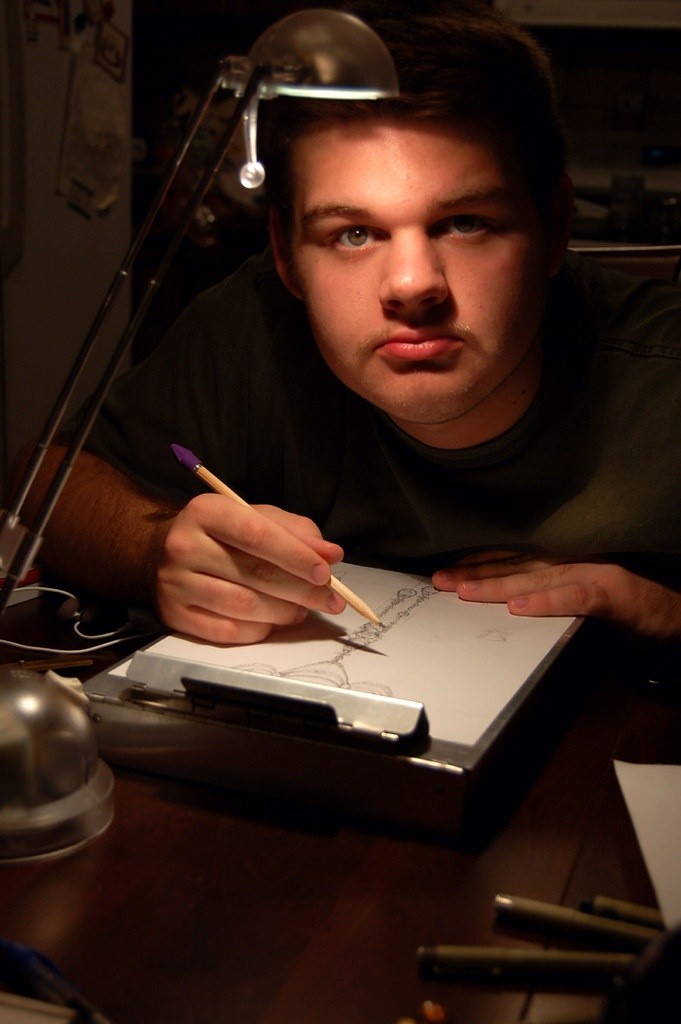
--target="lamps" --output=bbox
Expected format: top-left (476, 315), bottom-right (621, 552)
top-left (0, 8), bottom-right (401, 618)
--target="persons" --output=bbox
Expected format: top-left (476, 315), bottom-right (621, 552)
top-left (19, 14), bottom-right (681, 671)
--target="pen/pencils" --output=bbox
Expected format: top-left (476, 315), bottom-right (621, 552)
top-left (413, 941), bottom-right (636, 974)
top-left (168, 441), bottom-right (390, 631)
top-left (585, 896), bottom-right (666, 926)
top-left (493, 894), bottom-right (666, 947)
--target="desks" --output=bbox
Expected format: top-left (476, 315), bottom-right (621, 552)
top-left (0, 599), bottom-right (681, 1024)
